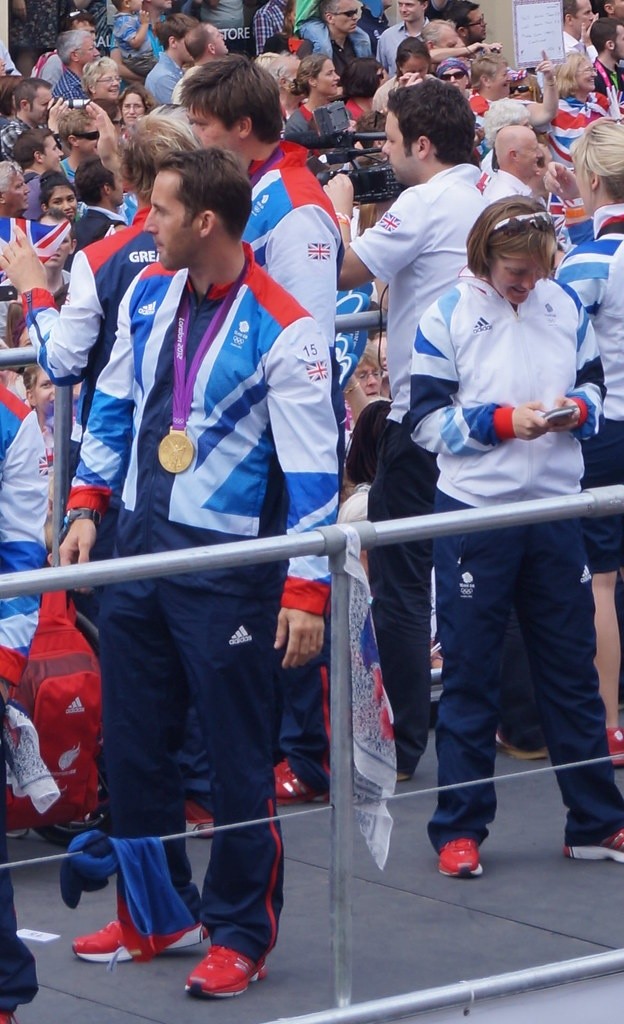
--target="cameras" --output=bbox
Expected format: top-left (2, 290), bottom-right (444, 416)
top-left (53, 97), bottom-right (91, 109)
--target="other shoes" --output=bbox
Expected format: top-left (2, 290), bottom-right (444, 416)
top-left (0, 1009), bottom-right (18, 1024)
top-left (496, 732), bottom-right (550, 759)
top-left (606, 726), bottom-right (624, 767)
top-left (396, 772), bottom-right (409, 781)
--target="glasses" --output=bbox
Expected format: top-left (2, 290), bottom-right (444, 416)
top-left (440, 71), bottom-right (466, 80)
top-left (510, 85), bottom-right (533, 94)
top-left (96, 77), bottom-right (122, 83)
top-left (487, 211), bottom-right (553, 237)
top-left (70, 131), bottom-right (99, 140)
top-left (466, 14), bottom-right (485, 28)
top-left (329, 6), bottom-right (358, 17)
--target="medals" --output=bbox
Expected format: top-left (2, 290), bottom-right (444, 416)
top-left (159, 428), bottom-right (194, 474)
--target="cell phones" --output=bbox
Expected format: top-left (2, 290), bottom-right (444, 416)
top-left (0, 285), bottom-right (18, 302)
top-left (539, 405), bottom-right (577, 422)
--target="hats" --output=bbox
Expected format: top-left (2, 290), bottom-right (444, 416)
top-left (436, 57), bottom-right (469, 81)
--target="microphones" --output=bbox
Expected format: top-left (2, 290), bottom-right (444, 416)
top-left (283, 131), bottom-right (340, 149)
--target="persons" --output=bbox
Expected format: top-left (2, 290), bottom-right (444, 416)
top-left (0, 0), bottom-right (624, 838)
top-left (47, 147), bottom-right (341, 998)
top-left (403, 195), bottom-right (624, 879)
top-left (0, 384), bottom-right (48, 1024)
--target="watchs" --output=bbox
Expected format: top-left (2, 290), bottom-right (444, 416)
top-left (65, 508), bottom-right (100, 526)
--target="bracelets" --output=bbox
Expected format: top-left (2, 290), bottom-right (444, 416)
top-left (336, 212), bottom-right (351, 225)
top-left (346, 383), bottom-right (360, 393)
top-left (546, 76), bottom-right (557, 87)
top-left (563, 198), bottom-right (584, 208)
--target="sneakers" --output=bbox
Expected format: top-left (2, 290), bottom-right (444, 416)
top-left (184, 800), bottom-right (213, 839)
top-left (274, 759), bottom-right (329, 805)
top-left (563, 828), bottom-right (624, 863)
top-left (438, 838), bottom-right (482, 878)
top-left (185, 945), bottom-right (268, 998)
top-left (72, 912), bottom-right (209, 973)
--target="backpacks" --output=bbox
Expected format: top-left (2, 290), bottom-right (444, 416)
top-left (5, 589), bottom-right (102, 832)
